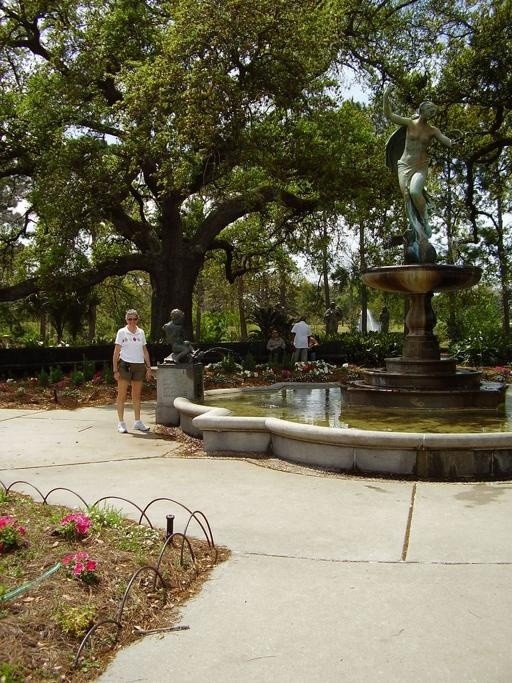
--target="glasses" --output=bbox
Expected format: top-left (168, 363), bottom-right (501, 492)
top-left (127, 317), bottom-right (137, 320)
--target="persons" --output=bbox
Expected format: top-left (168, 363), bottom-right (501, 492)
top-left (290, 317), bottom-right (312, 361)
top-left (323, 302), bottom-right (343, 335)
top-left (379, 306), bottom-right (390, 332)
top-left (309, 336), bottom-right (320, 361)
top-left (265, 330), bottom-right (286, 363)
top-left (383, 81), bottom-right (464, 239)
top-left (112, 308), bottom-right (152, 433)
top-left (162, 308), bottom-right (200, 365)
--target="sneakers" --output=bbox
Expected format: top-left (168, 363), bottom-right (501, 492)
top-left (117, 423), bottom-right (127, 433)
top-left (132, 421), bottom-right (150, 431)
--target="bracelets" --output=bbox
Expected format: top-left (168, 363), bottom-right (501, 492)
top-left (146, 367), bottom-right (151, 369)
top-left (113, 370), bottom-right (119, 374)
